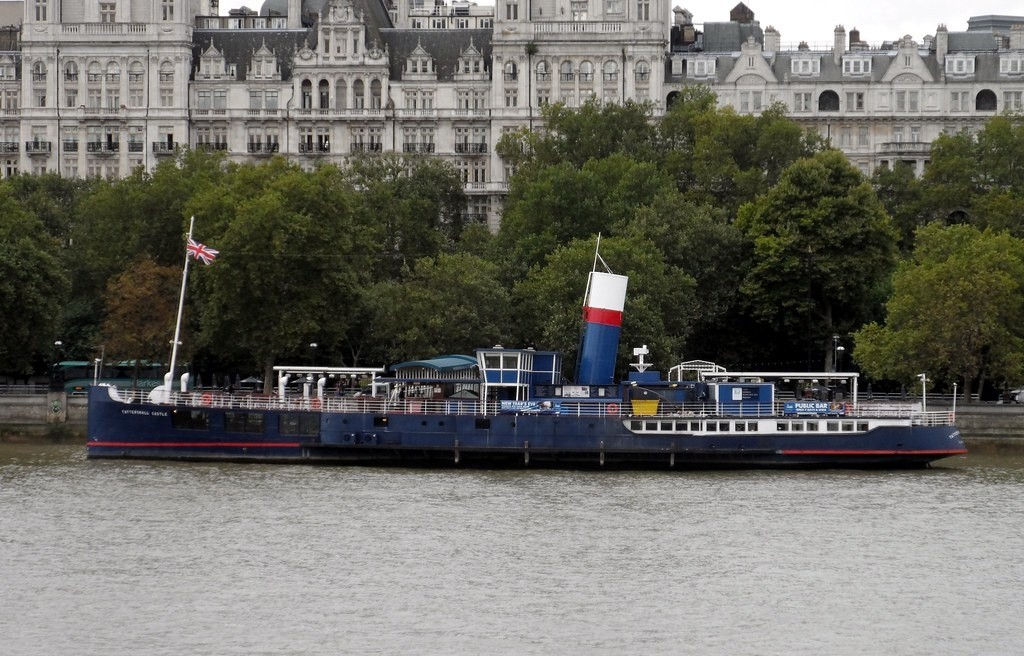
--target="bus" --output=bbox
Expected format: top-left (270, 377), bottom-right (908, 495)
top-left (44, 360), bottom-right (195, 397)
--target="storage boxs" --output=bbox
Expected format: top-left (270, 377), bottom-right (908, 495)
top-left (630, 399), bottom-right (659, 415)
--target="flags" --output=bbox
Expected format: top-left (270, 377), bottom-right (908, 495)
top-left (186, 238), bottom-right (219, 266)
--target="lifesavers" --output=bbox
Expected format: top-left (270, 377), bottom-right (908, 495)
top-left (311, 398), bottom-right (321, 409)
top-left (200, 393), bottom-right (212, 405)
top-left (607, 403), bottom-right (619, 415)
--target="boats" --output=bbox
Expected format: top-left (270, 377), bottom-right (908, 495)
top-left (87, 215), bottom-right (970, 469)
